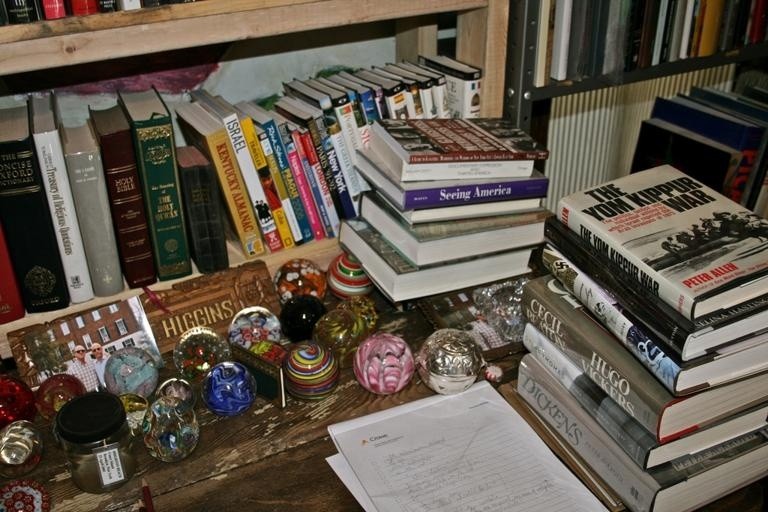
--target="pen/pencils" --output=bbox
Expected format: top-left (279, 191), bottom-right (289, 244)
top-left (142, 476), bottom-right (154, 512)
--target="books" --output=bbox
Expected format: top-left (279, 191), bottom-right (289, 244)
top-left (3, 1), bottom-right (768, 512)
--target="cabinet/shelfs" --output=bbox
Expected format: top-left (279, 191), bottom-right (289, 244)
top-left (1, 0), bottom-right (509, 357)
